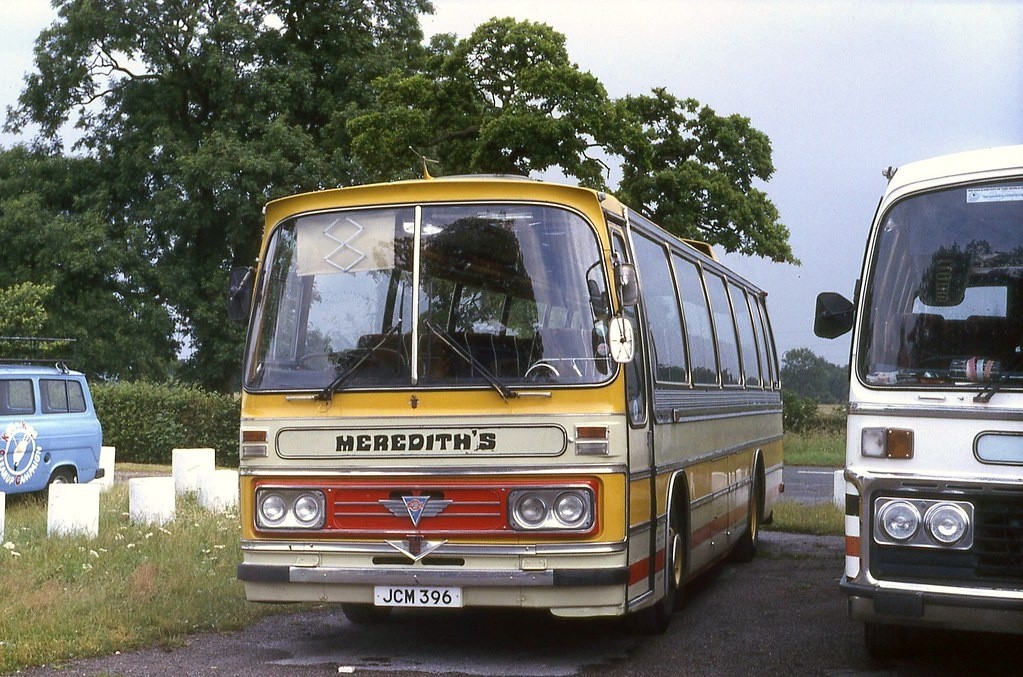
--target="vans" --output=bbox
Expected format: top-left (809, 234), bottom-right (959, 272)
top-left (0, 364), bottom-right (103, 503)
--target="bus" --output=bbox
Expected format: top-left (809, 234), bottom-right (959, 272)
top-left (814, 142), bottom-right (1023, 658)
top-left (227, 173), bottom-right (784, 637)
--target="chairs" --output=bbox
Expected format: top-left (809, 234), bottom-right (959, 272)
top-left (355, 328), bottom-right (589, 384)
top-left (887, 311), bottom-right (1020, 362)
